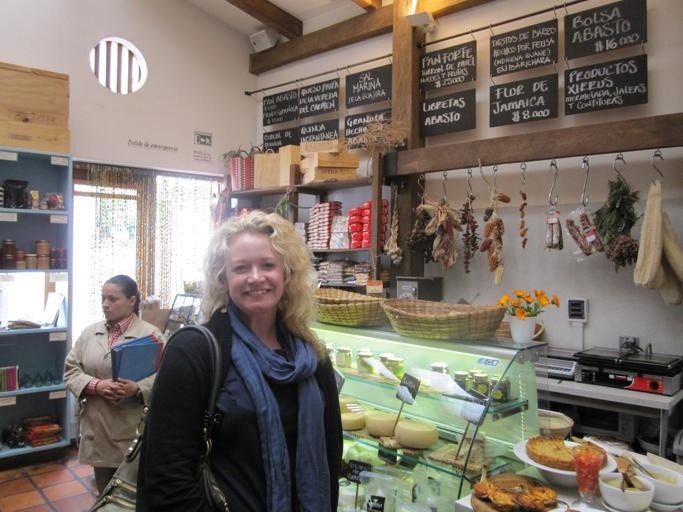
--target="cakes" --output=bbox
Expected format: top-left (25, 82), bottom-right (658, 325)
top-left (526, 435), bottom-right (607, 472)
top-left (474, 478), bottom-right (556, 511)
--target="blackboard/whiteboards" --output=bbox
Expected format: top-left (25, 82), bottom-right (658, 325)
top-left (346, 64), bottom-right (392, 108)
top-left (564, 55), bottom-right (647, 115)
top-left (490, 19), bottom-right (558, 77)
top-left (344, 108), bottom-right (391, 149)
top-left (565, 0), bottom-right (647, 61)
top-left (419, 40), bottom-right (477, 93)
top-left (263, 127), bottom-right (300, 153)
top-left (299, 78), bottom-right (339, 118)
top-left (420, 89), bottom-right (475, 138)
top-left (300, 118), bottom-right (339, 143)
top-left (263, 89), bottom-right (299, 126)
top-left (490, 73), bottom-right (558, 127)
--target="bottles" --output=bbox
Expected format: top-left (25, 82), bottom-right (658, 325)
top-left (0, 239), bottom-right (66, 271)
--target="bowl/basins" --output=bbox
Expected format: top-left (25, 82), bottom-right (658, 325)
top-left (538, 412), bottom-right (576, 437)
top-left (512, 437), bottom-right (618, 488)
top-left (597, 473), bottom-right (654, 512)
top-left (637, 463), bottom-right (682, 505)
top-left (636, 433), bottom-right (671, 458)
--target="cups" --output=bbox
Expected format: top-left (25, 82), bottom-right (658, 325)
top-left (617, 335), bottom-right (637, 354)
top-left (509, 317), bottom-right (544, 343)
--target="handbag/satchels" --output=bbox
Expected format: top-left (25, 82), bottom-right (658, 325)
top-left (88, 434), bottom-right (228, 512)
top-left (229, 146), bottom-right (280, 193)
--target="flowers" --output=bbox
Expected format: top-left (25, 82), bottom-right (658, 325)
top-left (498, 286), bottom-right (562, 321)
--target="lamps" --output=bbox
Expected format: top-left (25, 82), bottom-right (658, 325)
top-left (401, 6), bottom-right (440, 39)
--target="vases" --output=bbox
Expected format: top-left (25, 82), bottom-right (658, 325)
top-left (504, 312), bottom-right (545, 344)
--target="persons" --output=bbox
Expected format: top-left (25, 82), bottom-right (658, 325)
top-left (63, 274), bottom-right (168, 495)
top-left (136, 208), bottom-right (342, 512)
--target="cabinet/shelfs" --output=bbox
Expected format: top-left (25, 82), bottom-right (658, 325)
top-left (0, 142), bottom-right (83, 463)
top-left (304, 308), bottom-right (555, 511)
top-left (220, 149), bottom-right (397, 297)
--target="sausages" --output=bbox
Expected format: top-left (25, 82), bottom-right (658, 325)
top-left (544, 212), bottom-right (604, 256)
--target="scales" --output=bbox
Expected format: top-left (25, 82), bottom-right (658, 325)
top-left (534, 297), bottom-right (589, 381)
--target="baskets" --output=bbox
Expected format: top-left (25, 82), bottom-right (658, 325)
top-left (379, 297), bottom-right (508, 340)
top-left (311, 288), bottom-right (386, 327)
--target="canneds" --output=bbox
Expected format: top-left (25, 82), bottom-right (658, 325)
top-left (431, 362), bottom-right (510, 403)
top-left (2, 238), bottom-right (67, 270)
top-left (326, 342), bottom-right (403, 382)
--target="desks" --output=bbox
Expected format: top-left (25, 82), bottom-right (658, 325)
top-left (444, 445), bottom-right (683, 512)
top-left (526, 367), bottom-right (683, 462)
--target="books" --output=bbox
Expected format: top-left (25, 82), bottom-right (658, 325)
top-left (41, 292), bottom-right (65, 327)
top-left (7, 319), bottom-right (41, 330)
top-left (0, 364), bottom-right (19, 392)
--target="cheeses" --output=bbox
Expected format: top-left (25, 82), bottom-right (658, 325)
top-left (338, 397), bottom-right (438, 449)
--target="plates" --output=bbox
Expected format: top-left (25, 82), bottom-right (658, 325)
top-left (599, 496), bottom-right (650, 512)
top-left (651, 500), bottom-right (682, 512)
